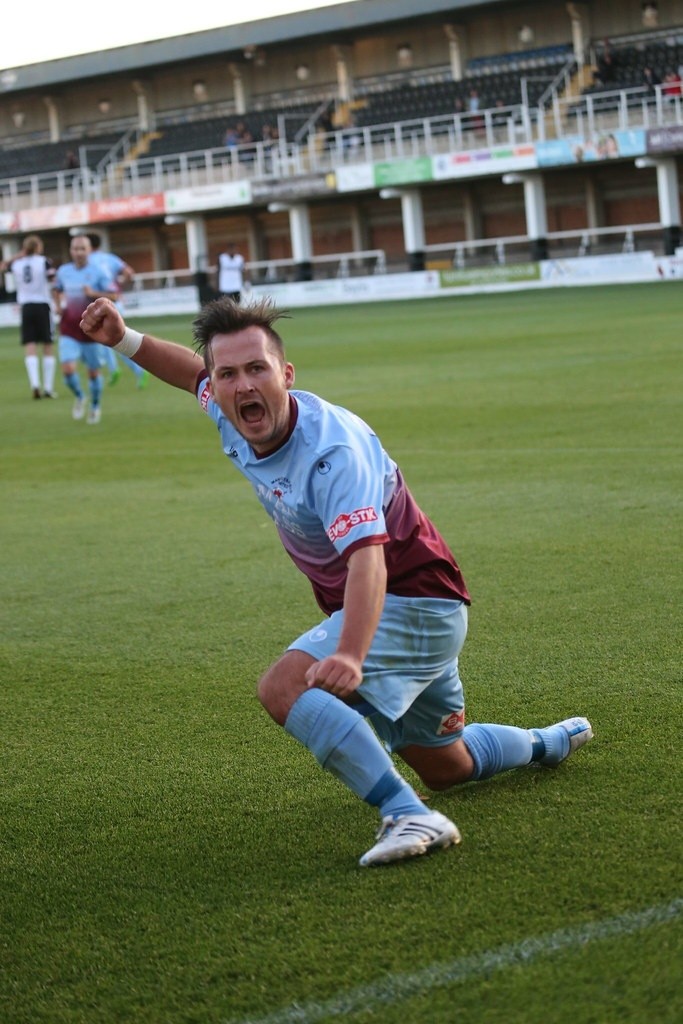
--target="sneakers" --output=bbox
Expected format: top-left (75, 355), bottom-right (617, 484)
top-left (525, 717), bottom-right (594, 768)
top-left (358, 809), bottom-right (462, 868)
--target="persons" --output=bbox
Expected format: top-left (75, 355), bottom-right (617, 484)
top-left (86, 233), bottom-right (152, 390)
top-left (80, 297), bottom-right (595, 867)
top-left (261, 123), bottom-right (279, 150)
top-left (0, 236), bottom-right (59, 399)
top-left (494, 100), bottom-right (510, 126)
top-left (217, 243), bottom-right (245, 302)
top-left (452, 87), bottom-right (486, 129)
top-left (663, 66), bottom-right (681, 94)
top-left (51, 234), bottom-right (121, 424)
top-left (641, 67), bottom-right (661, 86)
top-left (319, 106), bottom-right (332, 131)
top-left (593, 36), bottom-right (615, 90)
top-left (223, 121), bottom-right (255, 153)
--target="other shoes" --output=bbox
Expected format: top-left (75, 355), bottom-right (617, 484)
top-left (33, 388), bottom-right (40, 400)
top-left (87, 407), bottom-right (101, 425)
top-left (44, 390), bottom-right (56, 400)
top-left (72, 396), bottom-right (86, 420)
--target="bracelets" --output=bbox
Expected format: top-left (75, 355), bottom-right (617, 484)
top-left (112, 327), bottom-right (143, 359)
